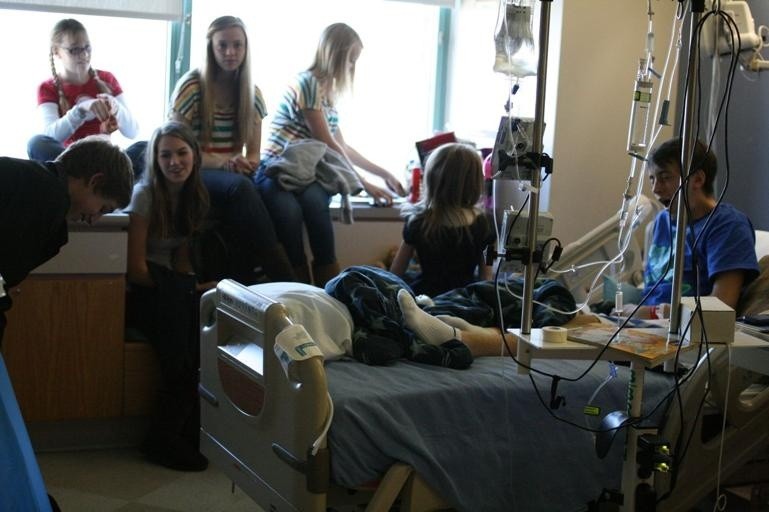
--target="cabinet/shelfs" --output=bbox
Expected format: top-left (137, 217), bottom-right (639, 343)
top-left (0, 225), bottom-right (125, 422)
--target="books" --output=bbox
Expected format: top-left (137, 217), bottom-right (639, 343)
top-left (565, 324), bottom-right (697, 366)
top-left (415, 132), bottom-right (459, 169)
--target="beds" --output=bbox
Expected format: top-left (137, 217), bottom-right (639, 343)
top-left (195, 278), bottom-right (769, 510)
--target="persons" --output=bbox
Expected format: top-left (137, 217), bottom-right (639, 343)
top-left (258, 23), bottom-right (406, 289)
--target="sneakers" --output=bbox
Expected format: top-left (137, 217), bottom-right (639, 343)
top-left (142, 428), bottom-right (209, 470)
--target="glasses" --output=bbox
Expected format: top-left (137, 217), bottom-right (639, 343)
top-left (55, 44), bottom-right (92, 55)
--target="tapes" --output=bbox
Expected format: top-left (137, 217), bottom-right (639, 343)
top-left (543, 326), bottom-right (567, 343)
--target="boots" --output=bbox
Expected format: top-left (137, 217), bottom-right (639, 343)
top-left (302, 253), bottom-right (339, 288)
